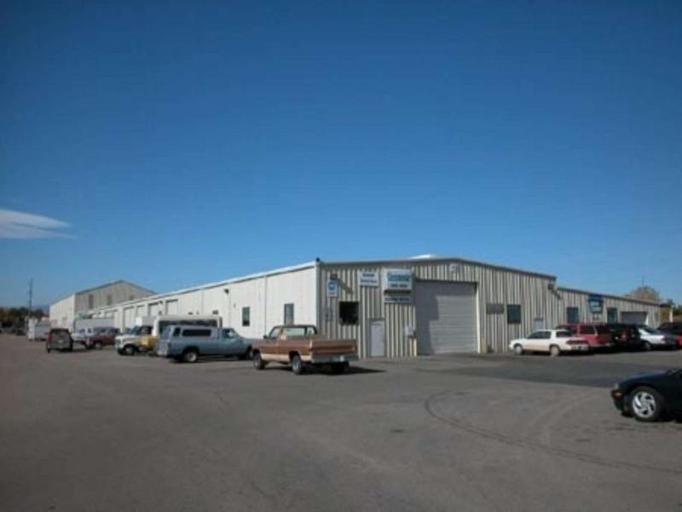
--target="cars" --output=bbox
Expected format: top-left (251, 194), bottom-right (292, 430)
top-left (612, 366), bottom-right (682, 423)
top-left (509, 322), bottom-right (682, 357)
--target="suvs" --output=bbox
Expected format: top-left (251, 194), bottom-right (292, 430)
top-left (46, 313), bottom-right (255, 361)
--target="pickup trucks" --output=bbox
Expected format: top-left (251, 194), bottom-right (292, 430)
top-left (250, 324), bottom-right (358, 373)
